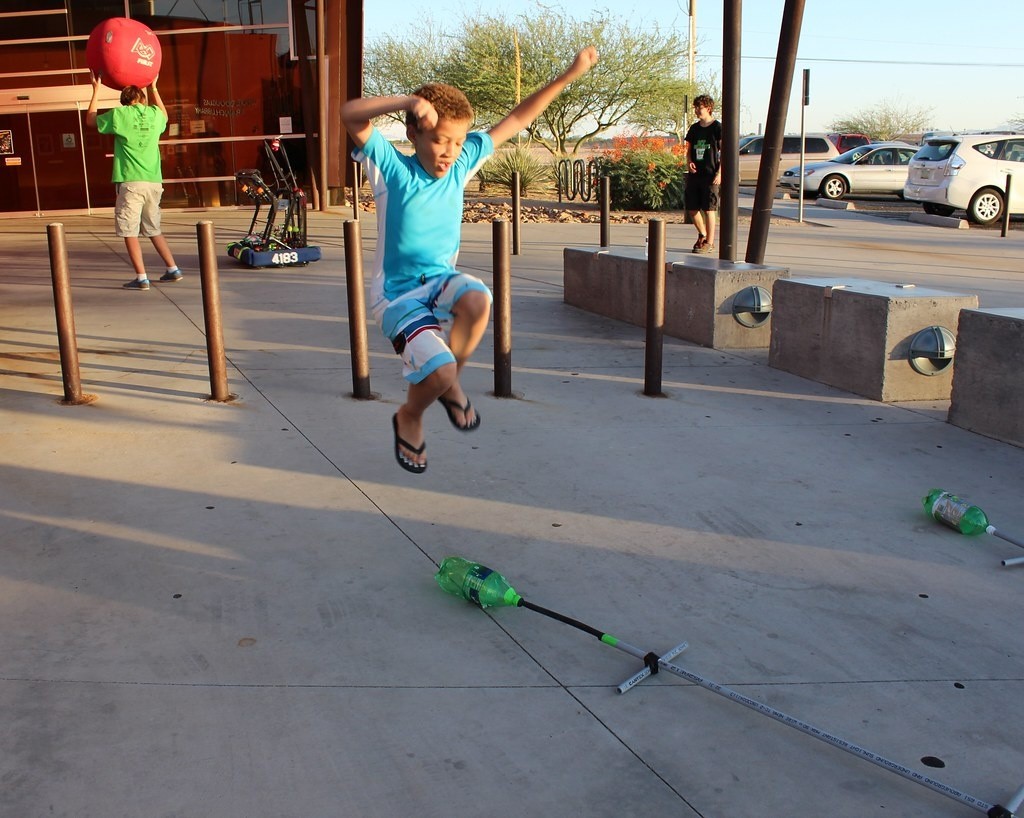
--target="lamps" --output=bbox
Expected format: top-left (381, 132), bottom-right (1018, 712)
top-left (732, 285), bottom-right (772, 328)
top-left (908, 325), bottom-right (956, 377)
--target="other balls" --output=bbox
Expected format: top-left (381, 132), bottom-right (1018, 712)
top-left (86, 16), bottom-right (163, 91)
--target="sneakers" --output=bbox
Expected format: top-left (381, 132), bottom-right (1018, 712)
top-left (160, 270), bottom-right (183, 282)
top-left (693, 233), bottom-right (708, 253)
top-left (123, 278), bottom-right (150, 291)
top-left (699, 243), bottom-right (715, 254)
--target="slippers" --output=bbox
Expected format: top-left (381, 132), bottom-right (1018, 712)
top-left (391, 411), bottom-right (428, 474)
top-left (439, 395), bottom-right (481, 430)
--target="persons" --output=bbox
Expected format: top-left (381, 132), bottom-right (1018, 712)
top-left (682, 95), bottom-right (722, 253)
top-left (340, 45), bottom-right (597, 467)
top-left (86, 69), bottom-right (183, 290)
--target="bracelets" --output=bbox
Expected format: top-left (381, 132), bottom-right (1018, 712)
top-left (150, 87), bottom-right (158, 93)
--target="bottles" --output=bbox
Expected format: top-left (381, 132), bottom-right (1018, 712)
top-left (435, 554), bottom-right (522, 611)
top-left (921, 487), bottom-right (993, 535)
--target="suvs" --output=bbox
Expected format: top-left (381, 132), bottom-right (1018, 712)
top-left (826, 133), bottom-right (871, 155)
top-left (714, 134), bottom-right (841, 185)
top-left (903, 135), bottom-right (1024, 225)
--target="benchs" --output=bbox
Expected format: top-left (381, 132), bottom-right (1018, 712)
top-left (945, 308), bottom-right (1024, 450)
top-left (561, 245), bottom-right (791, 353)
top-left (766, 274), bottom-right (979, 403)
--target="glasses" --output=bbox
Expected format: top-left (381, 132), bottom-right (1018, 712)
top-left (693, 106), bottom-right (707, 110)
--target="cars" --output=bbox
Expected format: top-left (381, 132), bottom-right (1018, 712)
top-left (778, 145), bottom-right (923, 204)
